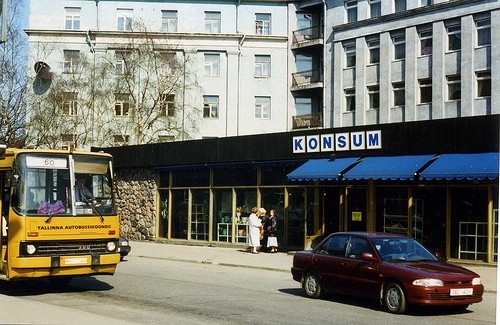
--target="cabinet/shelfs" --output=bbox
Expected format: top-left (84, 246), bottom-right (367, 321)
top-left (381, 196), bottom-right (424, 245)
top-left (458, 220), bottom-right (487, 262)
top-left (492, 207), bottom-right (499, 262)
top-left (179, 201), bottom-right (207, 240)
top-left (216, 222), bottom-right (248, 244)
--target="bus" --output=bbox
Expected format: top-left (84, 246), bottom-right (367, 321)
top-left (0, 145), bottom-right (121, 281)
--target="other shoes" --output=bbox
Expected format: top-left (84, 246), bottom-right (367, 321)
top-left (252, 251), bottom-right (259, 254)
top-left (268, 250), bottom-right (277, 253)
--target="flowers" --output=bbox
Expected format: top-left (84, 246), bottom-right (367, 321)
top-left (235, 206), bottom-right (245, 212)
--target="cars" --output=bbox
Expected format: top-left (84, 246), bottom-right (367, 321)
top-left (291, 232), bottom-right (484, 315)
top-left (118, 236), bottom-right (132, 260)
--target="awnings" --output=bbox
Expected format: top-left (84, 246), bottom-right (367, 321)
top-left (342, 154), bottom-right (436, 180)
top-left (286, 156), bottom-right (361, 181)
top-left (418, 152), bottom-right (500, 180)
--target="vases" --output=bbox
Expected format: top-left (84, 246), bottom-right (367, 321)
top-left (236, 211), bottom-right (241, 221)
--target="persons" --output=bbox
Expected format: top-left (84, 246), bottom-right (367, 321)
top-left (75, 179), bottom-right (94, 202)
top-left (249, 207), bottom-right (278, 254)
